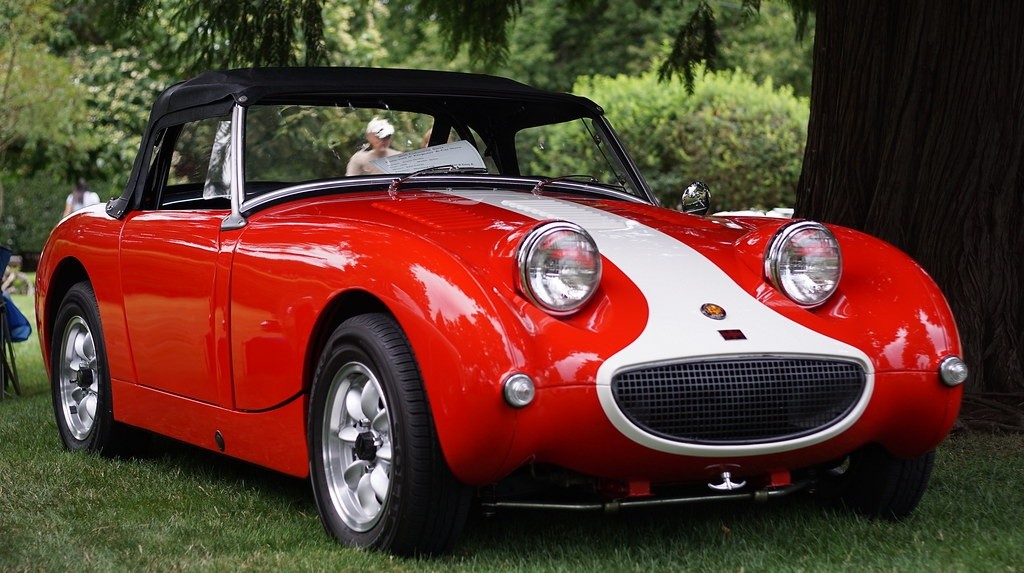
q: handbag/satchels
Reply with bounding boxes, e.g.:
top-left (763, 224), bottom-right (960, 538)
top-left (0, 292), bottom-right (32, 342)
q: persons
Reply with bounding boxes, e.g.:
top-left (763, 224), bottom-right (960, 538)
top-left (63, 177), bottom-right (100, 219)
top-left (344, 118), bottom-right (403, 176)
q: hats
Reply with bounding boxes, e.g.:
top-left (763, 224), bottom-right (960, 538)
top-left (365, 117), bottom-right (394, 139)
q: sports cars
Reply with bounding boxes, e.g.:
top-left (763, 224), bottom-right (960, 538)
top-left (32, 67), bottom-right (968, 562)
